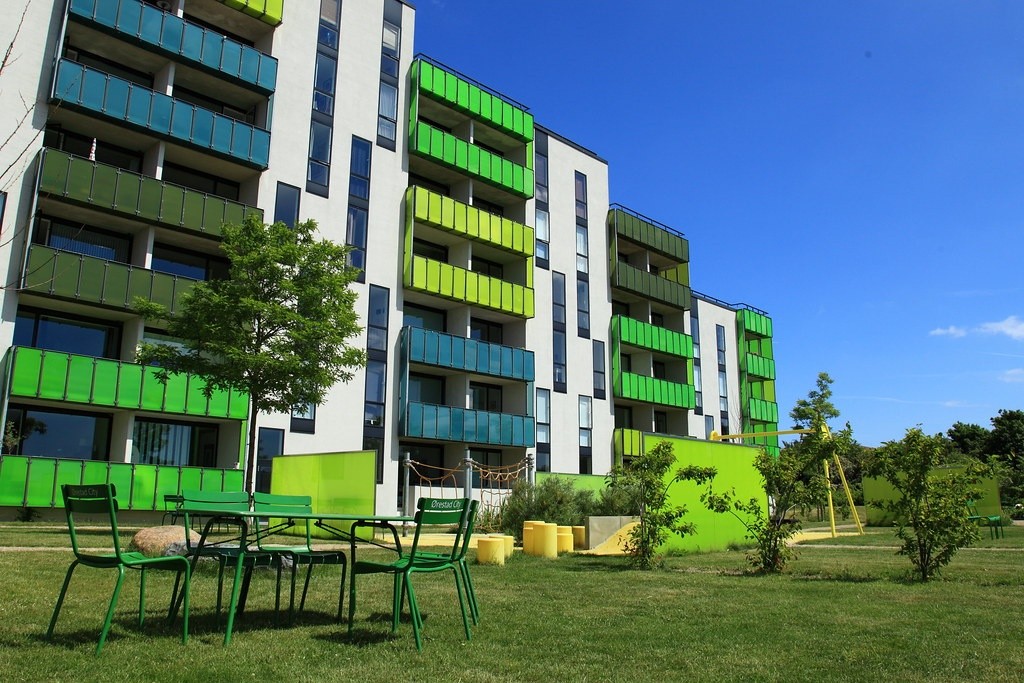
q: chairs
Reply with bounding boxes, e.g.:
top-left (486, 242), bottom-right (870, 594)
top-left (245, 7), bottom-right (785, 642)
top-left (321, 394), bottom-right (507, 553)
top-left (161, 495), bottom-right (194, 530)
top-left (347, 497), bottom-right (470, 654)
top-left (253, 492), bottom-right (346, 622)
top-left (167, 492), bottom-right (283, 625)
top-left (400, 500), bottom-right (479, 626)
top-left (46, 484), bottom-right (190, 659)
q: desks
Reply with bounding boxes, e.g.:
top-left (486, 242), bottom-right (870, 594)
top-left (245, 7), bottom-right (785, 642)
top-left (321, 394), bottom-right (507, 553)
top-left (175, 508), bottom-right (414, 647)
